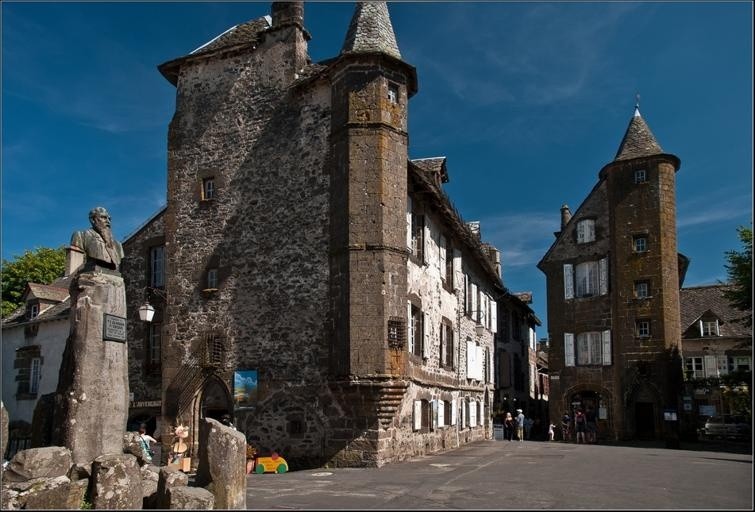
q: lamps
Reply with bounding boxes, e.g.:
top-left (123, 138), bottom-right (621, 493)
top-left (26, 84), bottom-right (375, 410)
top-left (137, 287), bottom-right (167, 323)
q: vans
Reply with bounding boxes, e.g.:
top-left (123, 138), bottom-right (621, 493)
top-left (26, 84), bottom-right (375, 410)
top-left (697, 412), bottom-right (753, 443)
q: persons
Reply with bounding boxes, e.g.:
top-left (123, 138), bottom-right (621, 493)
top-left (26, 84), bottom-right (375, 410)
top-left (560, 410), bottom-right (573, 442)
top-left (137, 428), bottom-right (157, 472)
top-left (523, 413), bottom-right (535, 441)
top-left (503, 411), bottom-right (512, 441)
top-left (547, 419), bottom-right (557, 441)
top-left (63, 204), bottom-right (129, 276)
top-left (221, 411), bottom-right (236, 428)
top-left (585, 406), bottom-right (599, 443)
top-left (514, 409), bottom-right (526, 443)
top-left (573, 408), bottom-right (588, 443)
